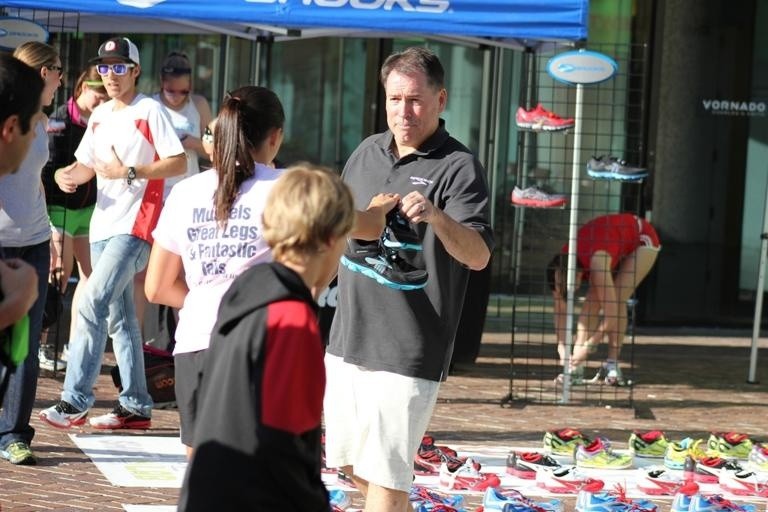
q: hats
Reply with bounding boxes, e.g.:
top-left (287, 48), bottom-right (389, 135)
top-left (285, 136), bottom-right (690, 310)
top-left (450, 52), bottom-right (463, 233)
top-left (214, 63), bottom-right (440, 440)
top-left (88, 37), bottom-right (140, 68)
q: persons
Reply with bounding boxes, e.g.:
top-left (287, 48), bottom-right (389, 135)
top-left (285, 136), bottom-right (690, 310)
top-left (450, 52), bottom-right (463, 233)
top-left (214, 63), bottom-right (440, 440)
top-left (546, 211), bottom-right (662, 384)
top-left (1, 36), bottom-right (219, 463)
top-left (176, 167), bottom-right (354, 511)
top-left (321, 47), bottom-right (495, 510)
top-left (142, 87), bottom-right (403, 460)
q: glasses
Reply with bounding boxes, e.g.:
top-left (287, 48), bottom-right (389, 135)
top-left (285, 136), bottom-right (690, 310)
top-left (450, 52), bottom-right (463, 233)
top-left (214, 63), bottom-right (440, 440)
top-left (95, 62), bottom-right (137, 77)
top-left (47, 65), bottom-right (63, 78)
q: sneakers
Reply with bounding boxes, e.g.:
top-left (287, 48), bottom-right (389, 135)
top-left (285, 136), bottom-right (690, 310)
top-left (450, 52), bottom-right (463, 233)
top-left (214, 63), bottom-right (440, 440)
top-left (586, 153), bottom-right (650, 183)
top-left (590, 482), bottom-right (657, 511)
top-left (483, 486), bottom-right (557, 512)
top-left (411, 435), bottom-right (500, 512)
top-left (544, 430), bottom-right (588, 456)
top-left (38, 401), bottom-right (88, 429)
top-left (379, 196), bottom-right (424, 252)
top-left (510, 184), bottom-right (570, 210)
top-left (338, 233), bottom-right (431, 292)
top-left (574, 437), bottom-right (633, 469)
top-left (550, 363), bottom-right (583, 388)
top-left (514, 101), bottom-right (576, 134)
top-left (89, 405), bottom-right (152, 429)
top-left (536, 466), bottom-right (605, 495)
top-left (627, 430), bottom-right (768, 509)
top-left (319, 429), bottom-right (365, 511)
top-left (0, 438), bottom-right (35, 467)
top-left (506, 450), bottom-right (562, 478)
top-left (599, 361), bottom-right (624, 386)
